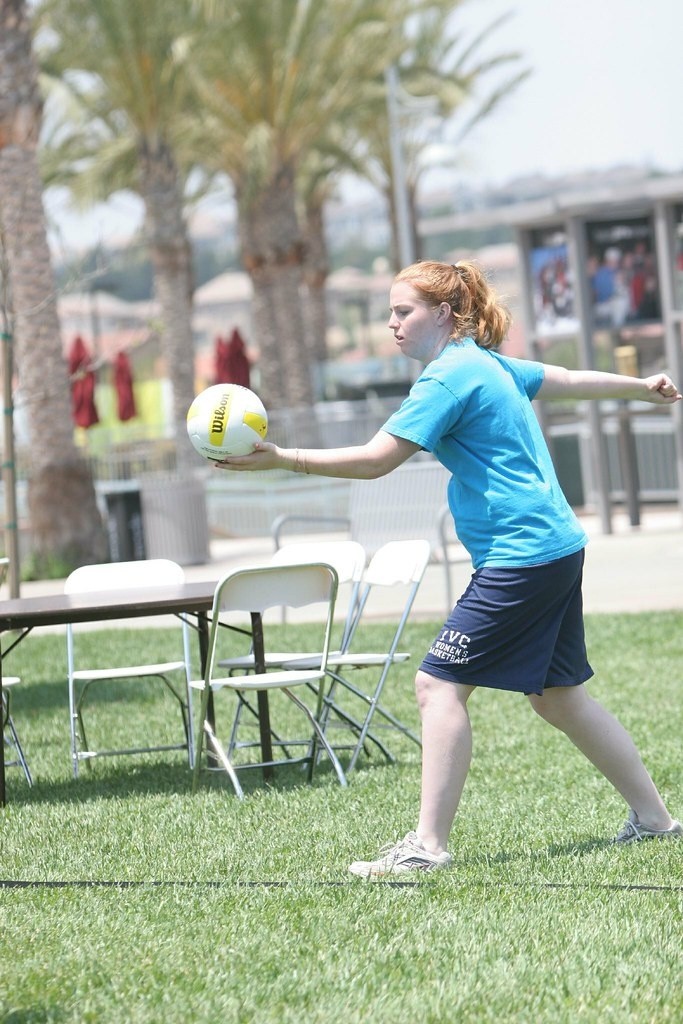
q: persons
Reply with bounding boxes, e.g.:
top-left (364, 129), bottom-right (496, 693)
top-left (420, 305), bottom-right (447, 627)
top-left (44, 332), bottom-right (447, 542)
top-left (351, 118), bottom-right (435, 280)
top-left (214, 260), bottom-right (683, 876)
top-left (586, 243), bottom-right (660, 328)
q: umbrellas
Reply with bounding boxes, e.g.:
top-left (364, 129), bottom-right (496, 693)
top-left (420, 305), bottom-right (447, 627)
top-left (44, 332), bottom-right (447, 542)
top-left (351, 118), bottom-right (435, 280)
top-left (67, 336), bottom-right (99, 447)
top-left (113, 353), bottom-right (136, 423)
top-left (214, 330), bottom-right (253, 389)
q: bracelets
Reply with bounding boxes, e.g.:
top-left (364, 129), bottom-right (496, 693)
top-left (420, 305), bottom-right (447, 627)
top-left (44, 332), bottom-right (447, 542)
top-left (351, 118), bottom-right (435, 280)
top-left (294, 447), bottom-right (310, 474)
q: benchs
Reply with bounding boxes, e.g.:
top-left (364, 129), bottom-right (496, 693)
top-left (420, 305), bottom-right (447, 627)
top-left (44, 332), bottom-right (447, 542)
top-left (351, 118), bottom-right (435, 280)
top-left (270, 461), bottom-right (458, 615)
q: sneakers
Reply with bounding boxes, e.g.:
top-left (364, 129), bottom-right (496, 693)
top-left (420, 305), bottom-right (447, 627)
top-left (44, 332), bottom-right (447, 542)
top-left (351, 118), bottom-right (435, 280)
top-left (347, 832), bottom-right (451, 877)
top-left (611, 808), bottom-right (683, 845)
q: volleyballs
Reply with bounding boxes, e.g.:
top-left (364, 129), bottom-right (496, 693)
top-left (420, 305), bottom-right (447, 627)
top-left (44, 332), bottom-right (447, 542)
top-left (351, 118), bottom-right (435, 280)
top-left (184, 383), bottom-right (271, 463)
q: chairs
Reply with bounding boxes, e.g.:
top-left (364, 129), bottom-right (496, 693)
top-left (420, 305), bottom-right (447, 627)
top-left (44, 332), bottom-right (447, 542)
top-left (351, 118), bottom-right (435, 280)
top-left (61, 538), bottom-right (431, 798)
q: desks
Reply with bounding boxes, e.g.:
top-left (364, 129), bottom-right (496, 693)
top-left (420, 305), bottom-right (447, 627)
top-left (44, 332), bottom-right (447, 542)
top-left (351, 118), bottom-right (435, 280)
top-left (0, 581), bottom-right (271, 807)
